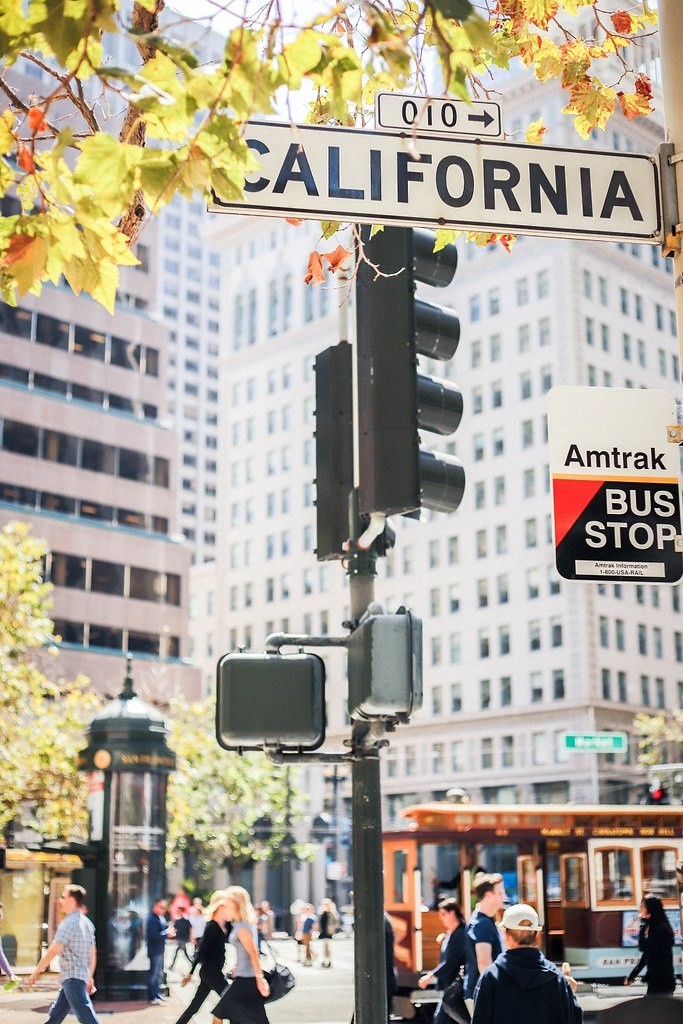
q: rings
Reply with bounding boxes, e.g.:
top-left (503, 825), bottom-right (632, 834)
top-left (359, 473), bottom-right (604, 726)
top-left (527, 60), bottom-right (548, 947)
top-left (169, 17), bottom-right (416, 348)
top-left (185, 977), bottom-right (186, 979)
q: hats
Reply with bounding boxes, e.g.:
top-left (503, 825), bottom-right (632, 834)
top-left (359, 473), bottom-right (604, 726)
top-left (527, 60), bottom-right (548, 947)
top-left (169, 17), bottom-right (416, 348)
top-left (498, 904), bottom-right (543, 931)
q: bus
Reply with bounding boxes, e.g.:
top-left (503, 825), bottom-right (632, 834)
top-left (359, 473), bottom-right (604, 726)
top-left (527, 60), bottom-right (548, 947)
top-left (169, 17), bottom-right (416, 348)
top-left (379, 807), bottom-right (683, 986)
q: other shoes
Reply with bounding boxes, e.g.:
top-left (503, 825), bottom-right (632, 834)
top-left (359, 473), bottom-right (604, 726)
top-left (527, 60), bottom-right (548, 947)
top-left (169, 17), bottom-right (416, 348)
top-left (151, 996), bottom-right (168, 1005)
top-left (321, 960), bottom-right (331, 968)
top-left (301, 959), bottom-right (312, 966)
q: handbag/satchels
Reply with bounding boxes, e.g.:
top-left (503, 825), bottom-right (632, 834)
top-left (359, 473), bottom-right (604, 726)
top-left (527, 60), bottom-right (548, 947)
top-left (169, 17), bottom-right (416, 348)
top-left (260, 963), bottom-right (296, 1004)
top-left (442, 977), bottom-right (471, 1024)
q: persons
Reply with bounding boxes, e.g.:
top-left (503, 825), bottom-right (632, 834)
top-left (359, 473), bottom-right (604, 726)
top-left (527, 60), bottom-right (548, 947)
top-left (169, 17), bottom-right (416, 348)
top-left (351, 909), bottom-right (398, 1024)
top-left (291, 898), bottom-right (354, 968)
top-left (30, 884), bottom-right (103, 1024)
top-left (417, 873), bottom-right (507, 1024)
top-left (146, 899), bottom-right (178, 1004)
top-left (471, 903), bottom-right (582, 1024)
top-left (624, 894), bottom-right (677, 996)
top-left (168, 886), bottom-right (276, 1024)
top-left (107, 909), bottom-right (144, 961)
top-left (0, 904), bottom-right (24, 983)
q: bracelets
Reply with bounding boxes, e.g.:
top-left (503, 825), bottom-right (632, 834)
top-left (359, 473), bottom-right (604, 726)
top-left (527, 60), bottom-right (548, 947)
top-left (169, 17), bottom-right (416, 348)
top-left (253, 974), bottom-right (265, 978)
top-left (426, 974), bottom-right (433, 979)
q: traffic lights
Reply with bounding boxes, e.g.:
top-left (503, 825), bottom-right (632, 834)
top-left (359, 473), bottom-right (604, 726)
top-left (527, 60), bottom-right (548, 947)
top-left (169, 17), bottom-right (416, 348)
top-left (347, 605), bottom-right (425, 722)
top-left (647, 780), bottom-right (665, 806)
top-left (355, 226), bottom-right (466, 519)
